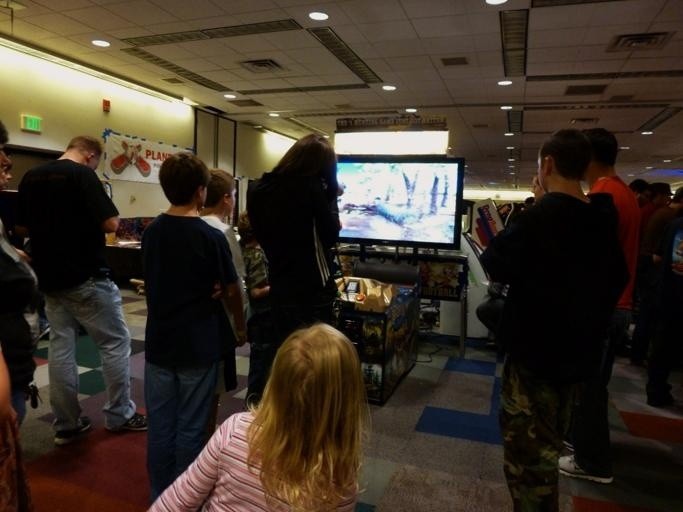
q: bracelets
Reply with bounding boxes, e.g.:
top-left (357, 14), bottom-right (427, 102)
top-left (236, 324), bottom-right (247, 338)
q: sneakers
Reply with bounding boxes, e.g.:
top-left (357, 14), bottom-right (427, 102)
top-left (646, 390), bottom-right (683, 410)
top-left (103, 412), bottom-right (147, 432)
top-left (51, 415), bottom-right (97, 446)
top-left (558, 453), bottom-right (615, 484)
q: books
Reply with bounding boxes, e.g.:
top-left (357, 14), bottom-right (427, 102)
top-left (463, 198), bottom-right (506, 283)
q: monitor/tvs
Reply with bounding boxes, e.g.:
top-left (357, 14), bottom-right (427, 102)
top-left (336, 155), bottom-right (465, 250)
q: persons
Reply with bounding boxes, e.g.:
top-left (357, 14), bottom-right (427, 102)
top-left (139, 152), bottom-right (248, 502)
top-left (197, 167), bottom-right (249, 446)
top-left (238, 133), bottom-right (343, 411)
top-left (16, 136), bottom-right (147, 446)
top-left (0, 118), bottom-right (46, 512)
top-left (234, 210), bottom-right (270, 342)
top-left (479, 128), bottom-right (683, 512)
top-left (147, 321), bottom-right (364, 510)
